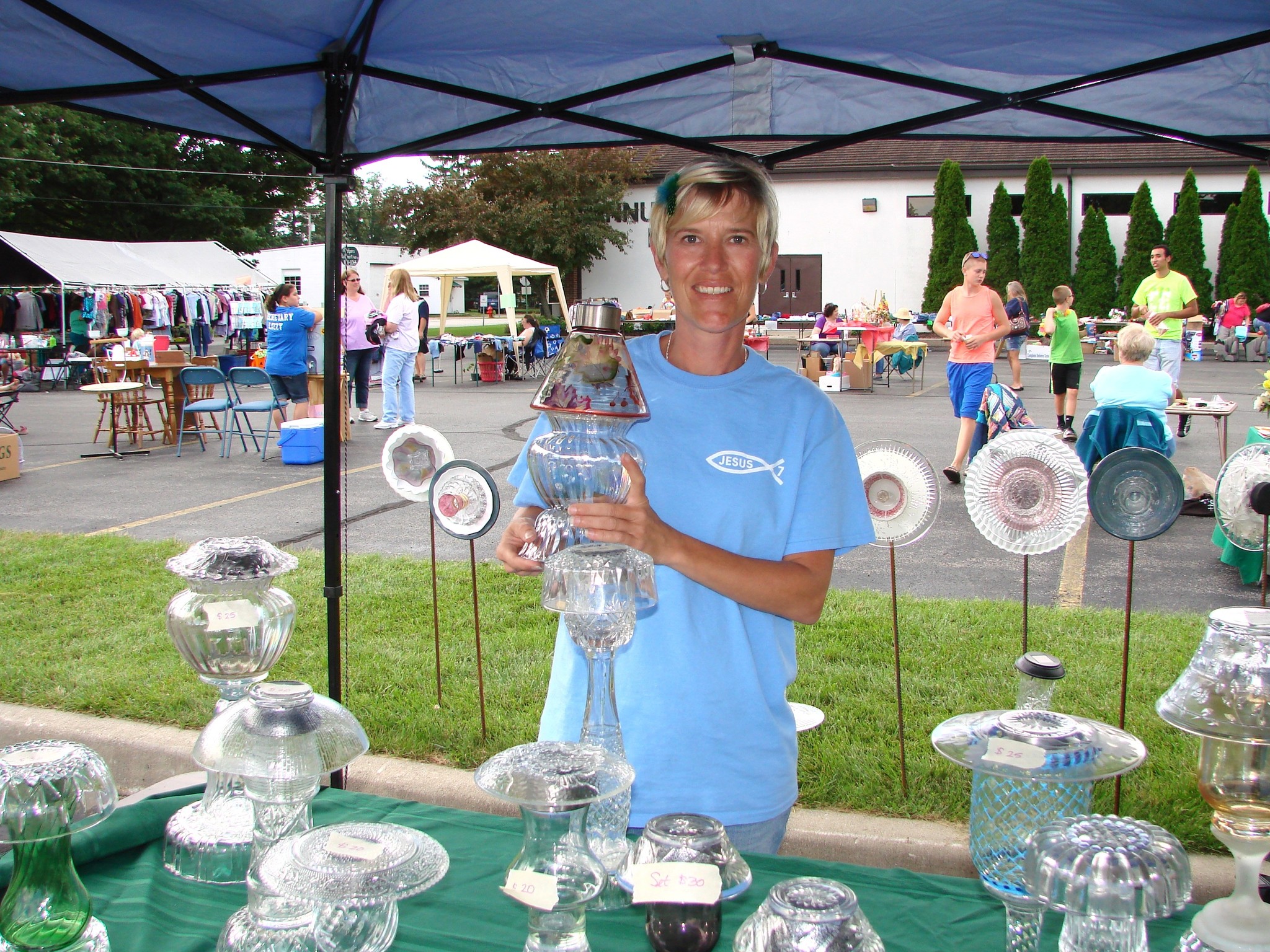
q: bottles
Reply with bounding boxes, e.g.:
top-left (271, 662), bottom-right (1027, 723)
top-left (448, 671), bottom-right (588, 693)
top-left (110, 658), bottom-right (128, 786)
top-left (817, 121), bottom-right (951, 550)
top-left (504, 301), bottom-right (719, 951)
top-left (964, 731), bottom-right (1270, 952)
top-left (165, 543), bottom-right (398, 952)
top-left (10, 336), bottom-right (16, 349)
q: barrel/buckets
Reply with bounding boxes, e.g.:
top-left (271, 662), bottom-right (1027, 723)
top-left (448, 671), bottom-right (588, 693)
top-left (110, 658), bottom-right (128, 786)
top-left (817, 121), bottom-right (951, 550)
top-left (23, 335), bottom-right (37, 348)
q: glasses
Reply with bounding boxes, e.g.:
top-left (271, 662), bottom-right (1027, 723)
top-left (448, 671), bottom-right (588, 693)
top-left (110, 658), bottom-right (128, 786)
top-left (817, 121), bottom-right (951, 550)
top-left (1065, 293), bottom-right (1075, 300)
top-left (963, 252), bottom-right (988, 267)
top-left (342, 278), bottom-right (360, 282)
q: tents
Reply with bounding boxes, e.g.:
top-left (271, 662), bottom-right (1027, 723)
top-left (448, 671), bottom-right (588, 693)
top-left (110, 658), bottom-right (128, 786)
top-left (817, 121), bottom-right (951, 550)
top-left (382, 239), bottom-right (575, 382)
top-left (1, 2), bottom-right (1266, 822)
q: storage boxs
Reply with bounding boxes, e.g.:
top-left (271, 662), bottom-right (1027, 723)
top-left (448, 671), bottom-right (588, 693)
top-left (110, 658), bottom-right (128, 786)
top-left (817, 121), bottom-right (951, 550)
top-left (622, 304), bottom-right (677, 321)
top-left (279, 416), bottom-right (329, 467)
top-left (0, 433), bottom-right (22, 484)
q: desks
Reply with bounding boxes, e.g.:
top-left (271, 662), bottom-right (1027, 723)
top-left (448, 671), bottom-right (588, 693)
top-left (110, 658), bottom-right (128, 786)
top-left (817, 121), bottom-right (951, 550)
top-left (431, 334), bottom-right (550, 386)
top-left (1025, 309), bottom-right (1267, 365)
top-left (13, 765), bottom-right (1270, 952)
top-left (1161, 399), bottom-right (1237, 465)
top-left (744, 311), bottom-right (952, 392)
top-left (302, 372), bottom-right (351, 445)
top-left (1214, 426), bottom-right (1270, 588)
top-left (620, 320), bottom-right (675, 336)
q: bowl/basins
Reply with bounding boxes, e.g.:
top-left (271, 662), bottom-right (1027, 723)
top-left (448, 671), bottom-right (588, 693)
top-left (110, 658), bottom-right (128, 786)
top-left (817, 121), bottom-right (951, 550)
top-left (1157, 606), bottom-right (1269, 748)
top-left (731, 872), bottom-right (885, 952)
top-left (614, 816), bottom-right (751, 900)
top-left (190, 682), bottom-right (367, 778)
top-left (117, 328), bottom-right (128, 337)
top-left (0, 738), bottom-right (117, 842)
top-left (88, 330), bottom-right (100, 338)
top-left (1022, 813), bottom-right (1195, 920)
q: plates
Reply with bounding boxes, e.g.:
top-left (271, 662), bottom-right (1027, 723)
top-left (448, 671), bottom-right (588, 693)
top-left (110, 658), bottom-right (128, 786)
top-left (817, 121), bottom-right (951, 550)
top-left (856, 437), bottom-right (940, 546)
top-left (1088, 446), bottom-right (1183, 539)
top-left (475, 741), bottom-right (637, 804)
top-left (963, 431), bottom-right (1089, 555)
top-left (382, 424), bottom-right (454, 503)
top-left (261, 818), bottom-right (451, 911)
top-left (426, 459), bottom-right (502, 540)
top-left (164, 535), bottom-right (298, 581)
top-left (927, 705), bottom-right (1144, 782)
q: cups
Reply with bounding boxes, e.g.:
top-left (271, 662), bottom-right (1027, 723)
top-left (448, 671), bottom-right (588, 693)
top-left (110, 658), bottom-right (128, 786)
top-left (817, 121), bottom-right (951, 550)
top-left (963, 335), bottom-right (973, 352)
top-left (1085, 322), bottom-right (1094, 338)
top-left (0, 771), bottom-right (90, 949)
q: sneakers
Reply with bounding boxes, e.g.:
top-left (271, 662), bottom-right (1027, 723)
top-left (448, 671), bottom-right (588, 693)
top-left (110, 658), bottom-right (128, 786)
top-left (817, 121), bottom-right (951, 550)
top-left (1063, 426), bottom-right (1077, 441)
top-left (1057, 423), bottom-right (1065, 431)
top-left (350, 417), bottom-right (355, 423)
top-left (373, 419), bottom-right (398, 429)
top-left (1176, 414), bottom-right (1191, 437)
top-left (397, 417), bottom-right (415, 426)
top-left (358, 409), bottom-right (378, 421)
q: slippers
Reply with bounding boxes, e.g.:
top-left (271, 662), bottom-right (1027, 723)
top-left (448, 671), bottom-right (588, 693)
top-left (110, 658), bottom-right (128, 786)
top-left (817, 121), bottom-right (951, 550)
top-left (963, 466), bottom-right (969, 476)
top-left (943, 466), bottom-right (961, 483)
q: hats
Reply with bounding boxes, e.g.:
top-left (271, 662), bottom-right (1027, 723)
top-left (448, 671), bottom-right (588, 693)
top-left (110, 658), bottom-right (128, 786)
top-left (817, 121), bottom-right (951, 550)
top-left (892, 307), bottom-right (914, 320)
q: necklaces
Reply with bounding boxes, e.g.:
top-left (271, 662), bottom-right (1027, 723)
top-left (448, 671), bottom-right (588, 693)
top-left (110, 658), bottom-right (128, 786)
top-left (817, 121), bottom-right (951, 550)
top-left (665, 330), bottom-right (748, 373)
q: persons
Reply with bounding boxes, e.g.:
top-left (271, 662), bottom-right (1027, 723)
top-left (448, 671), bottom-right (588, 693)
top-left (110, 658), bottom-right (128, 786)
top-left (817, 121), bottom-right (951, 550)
top-left (1212, 291), bottom-right (1251, 359)
top-left (340, 269), bottom-right (382, 425)
top-left (410, 287), bottom-right (431, 379)
top-left (263, 283), bottom-right (323, 434)
top-left (998, 280), bottom-right (1031, 390)
top-left (0, 336), bottom-right (11, 386)
top-left (68, 298), bottom-right (92, 384)
top-left (870, 309), bottom-right (918, 380)
top-left (504, 313), bottom-right (540, 377)
top-left (1038, 285), bottom-right (1097, 439)
top-left (379, 269), bottom-right (421, 428)
top-left (660, 292), bottom-right (674, 309)
top-left (809, 303), bottom-right (849, 361)
top-left (1089, 323), bottom-right (1179, 458)
top-left (499, 159), bottom-right (880, 854)
top-left (746, 302), bottom-right (757, 326)
top-left (1130, 245), bottom-right (1201, 437)
top-left (1253, 304), bottom-right (1270, 359)
top-left (929, 253), bottom-right (1011, 485)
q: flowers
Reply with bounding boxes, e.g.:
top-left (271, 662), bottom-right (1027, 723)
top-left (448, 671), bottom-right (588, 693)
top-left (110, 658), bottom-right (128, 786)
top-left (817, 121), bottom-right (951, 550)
top-left (1251, 368), bottom-right (1270, 420)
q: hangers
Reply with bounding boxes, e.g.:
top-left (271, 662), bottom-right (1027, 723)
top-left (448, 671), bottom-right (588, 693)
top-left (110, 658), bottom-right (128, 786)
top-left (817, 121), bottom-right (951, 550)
top-left (0, 284), bottom-right (277, 301)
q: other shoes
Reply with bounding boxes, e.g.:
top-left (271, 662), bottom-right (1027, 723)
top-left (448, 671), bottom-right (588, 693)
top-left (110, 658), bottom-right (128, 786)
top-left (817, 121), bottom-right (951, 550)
top-left (873, 374), bottom-right (882, 380)
top-left (413, 374), bottom-right (420, 377)
top-left (420, 374), bottom-right (427, 379)
top-left (1021, 387), bottom-right (1024, 390)
top-left (1008, 386), bottom-right (1021, 391)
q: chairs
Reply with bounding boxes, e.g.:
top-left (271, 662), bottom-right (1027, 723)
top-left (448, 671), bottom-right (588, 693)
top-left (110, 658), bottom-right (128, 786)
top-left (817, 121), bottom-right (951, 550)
top-left (0, 334), bottom-right (221, 459)
top-left (538, 325), bottom-right (564, 370)
top-left (1082, 405), bottom-right (1168, 470)
top-left (175, 366), bottom-right (248, 458)
top-left (223, 366), bottom-right (290, 462)
top-left (981, 384), bottom-right (1063, 440)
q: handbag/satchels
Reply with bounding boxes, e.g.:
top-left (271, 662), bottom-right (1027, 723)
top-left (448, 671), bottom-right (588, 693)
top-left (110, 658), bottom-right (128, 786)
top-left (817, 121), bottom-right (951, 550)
top-left (1009, 297), bottom-right (1028, 334)
